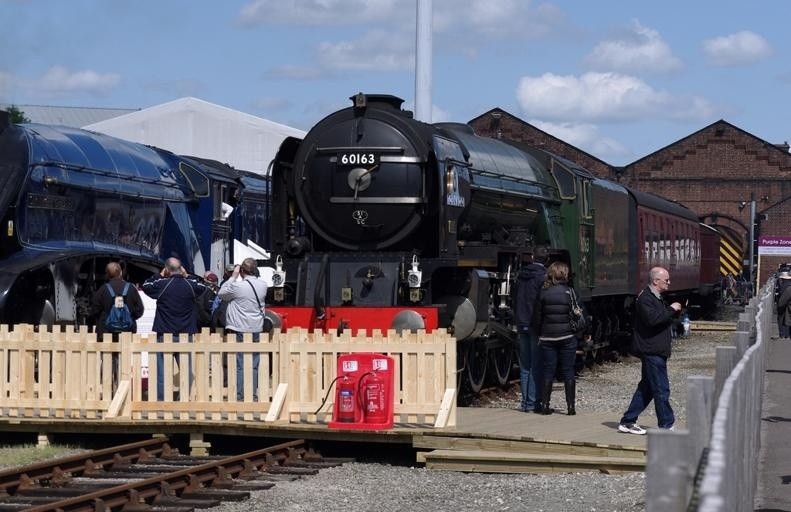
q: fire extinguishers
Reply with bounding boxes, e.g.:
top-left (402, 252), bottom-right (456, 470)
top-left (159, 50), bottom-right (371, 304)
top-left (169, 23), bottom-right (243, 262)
top-left (338, 367), bottom-right (388, 423)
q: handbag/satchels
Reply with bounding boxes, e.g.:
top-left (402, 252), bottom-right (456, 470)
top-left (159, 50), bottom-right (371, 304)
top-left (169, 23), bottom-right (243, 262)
top-left (569, 309), bottom-right (592, 333)
top-left (263, 316), bottom-right (273, 333)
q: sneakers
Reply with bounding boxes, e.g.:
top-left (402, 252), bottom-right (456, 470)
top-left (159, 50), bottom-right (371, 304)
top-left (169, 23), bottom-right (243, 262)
top-left (618, 422), bottom-right (647, 434)
top-left (522, 408), bottom-right (540, 413)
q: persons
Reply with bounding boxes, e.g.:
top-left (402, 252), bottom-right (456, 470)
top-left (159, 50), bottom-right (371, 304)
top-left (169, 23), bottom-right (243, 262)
top-left (772, 263), bottom-right (791, 340)
top-left (93, 261), bottom-right (146, 388)
top-left (718, 269), bottom-right (759, 308)
top-left (616, 266), bottom-right (684, 436)
top-left (512, 245), bottom-right (555, 411)
top-left (197, 257), bottom-right (270, 404)
top-left (530, 259), bottom-right (595, 415)
top-left (142, 256), bottom-right (212, 401)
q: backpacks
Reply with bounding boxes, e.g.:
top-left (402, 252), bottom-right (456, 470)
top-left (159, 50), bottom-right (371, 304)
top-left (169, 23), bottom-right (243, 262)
top-left (98, 282), bottom-right (133, 341)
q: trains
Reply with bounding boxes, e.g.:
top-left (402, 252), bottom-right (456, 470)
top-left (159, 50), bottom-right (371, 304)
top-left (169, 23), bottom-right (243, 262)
top-left (264, 90), bottom-right (746, 407)
top-left (2, 120), bottom-right (271, 333)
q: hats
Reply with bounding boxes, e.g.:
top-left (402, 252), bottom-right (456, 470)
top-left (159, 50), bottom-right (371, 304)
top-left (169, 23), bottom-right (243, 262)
top-left (207, 273), bottom-right (218, 282)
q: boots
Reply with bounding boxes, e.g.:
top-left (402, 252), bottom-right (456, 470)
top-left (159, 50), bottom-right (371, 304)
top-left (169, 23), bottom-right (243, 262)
top-left (541, 378), bottom-right (555, 415)
top-left (565, 379), bottom-right (576, 415)
